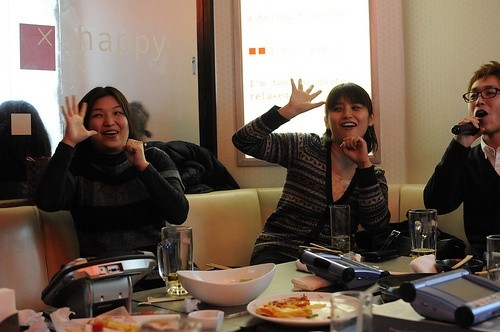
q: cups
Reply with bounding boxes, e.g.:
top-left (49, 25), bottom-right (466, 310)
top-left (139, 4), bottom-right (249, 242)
top-left (330, 291), bottom-right (373, 332)
top-left (408, 209), bottom-right (437, 262)
top-left (330, 205), bottom-right (351, 253)
top-left (486, 235), bottom-right (500, 283)
top-left (156, 226), bottom-right (194, 296)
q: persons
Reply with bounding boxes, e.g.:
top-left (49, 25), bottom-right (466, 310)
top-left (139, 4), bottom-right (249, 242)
top-left (422, 62), bottom-right (500, 265)
top-left (34, 87), bottom-right (199, 293)
top-left (232, 76), bottom-right (391, 266)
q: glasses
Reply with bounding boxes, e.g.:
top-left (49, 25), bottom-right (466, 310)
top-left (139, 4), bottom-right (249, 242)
top-left (462, 87), bottom-right (500, 104)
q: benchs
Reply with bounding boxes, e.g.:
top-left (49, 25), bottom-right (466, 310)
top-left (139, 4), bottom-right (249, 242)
top-left (0, 184), bottom-right (468, 312)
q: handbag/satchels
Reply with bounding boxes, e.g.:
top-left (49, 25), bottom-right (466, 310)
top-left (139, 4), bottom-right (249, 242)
top-left (350, 217), bottom-right (468, 263)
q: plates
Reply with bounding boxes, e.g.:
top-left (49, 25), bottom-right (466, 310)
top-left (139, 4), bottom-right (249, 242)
top-left (246, 291), bottom-right (360, 326)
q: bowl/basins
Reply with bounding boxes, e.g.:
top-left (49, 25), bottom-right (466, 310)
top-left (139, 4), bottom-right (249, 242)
top-left (434, 258), bottom-right (484, 275)
top-left (188, 309), bottom-right (224, 332)
top-left (377, 272), bottom-right (437, 304)
top-left (176, 262), bottom-right (276, 307)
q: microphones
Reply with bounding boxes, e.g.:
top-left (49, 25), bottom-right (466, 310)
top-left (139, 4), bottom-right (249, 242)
top-left (452, 122), bottom-right (480, 136)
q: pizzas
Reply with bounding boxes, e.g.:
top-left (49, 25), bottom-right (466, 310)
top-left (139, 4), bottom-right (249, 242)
top-left (256, 294), bottom-right (312, 317)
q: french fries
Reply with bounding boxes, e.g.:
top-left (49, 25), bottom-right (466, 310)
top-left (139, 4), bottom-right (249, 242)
top-left (87, 318), bottom-right (140, 332)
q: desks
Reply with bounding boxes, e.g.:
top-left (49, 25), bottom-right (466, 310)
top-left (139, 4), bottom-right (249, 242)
top-left (44, 253), bottom-right (500, 332)
